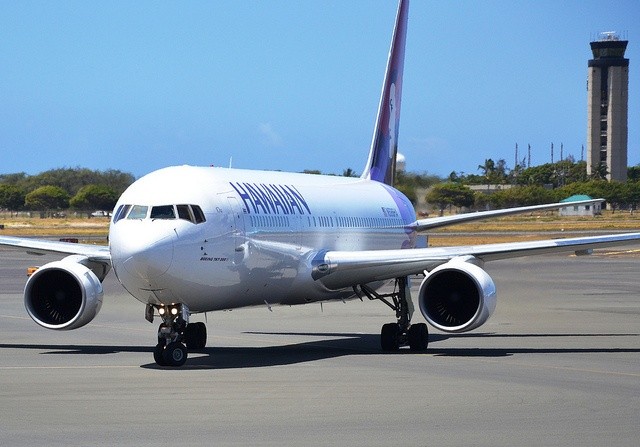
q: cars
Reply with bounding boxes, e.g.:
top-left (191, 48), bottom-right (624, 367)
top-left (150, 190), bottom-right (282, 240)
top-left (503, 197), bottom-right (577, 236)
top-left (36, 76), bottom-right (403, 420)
top-left (14, 210), bottom-right (29, 215)
top-left (90, 209), bottom-right (111, 216)
top-left (40, 210), bottom-right (65, 217)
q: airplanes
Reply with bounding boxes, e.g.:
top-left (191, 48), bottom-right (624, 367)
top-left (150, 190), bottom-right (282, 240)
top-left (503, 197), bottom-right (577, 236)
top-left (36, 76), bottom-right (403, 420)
top-left (0, 0), bottom-right (639, 365)
